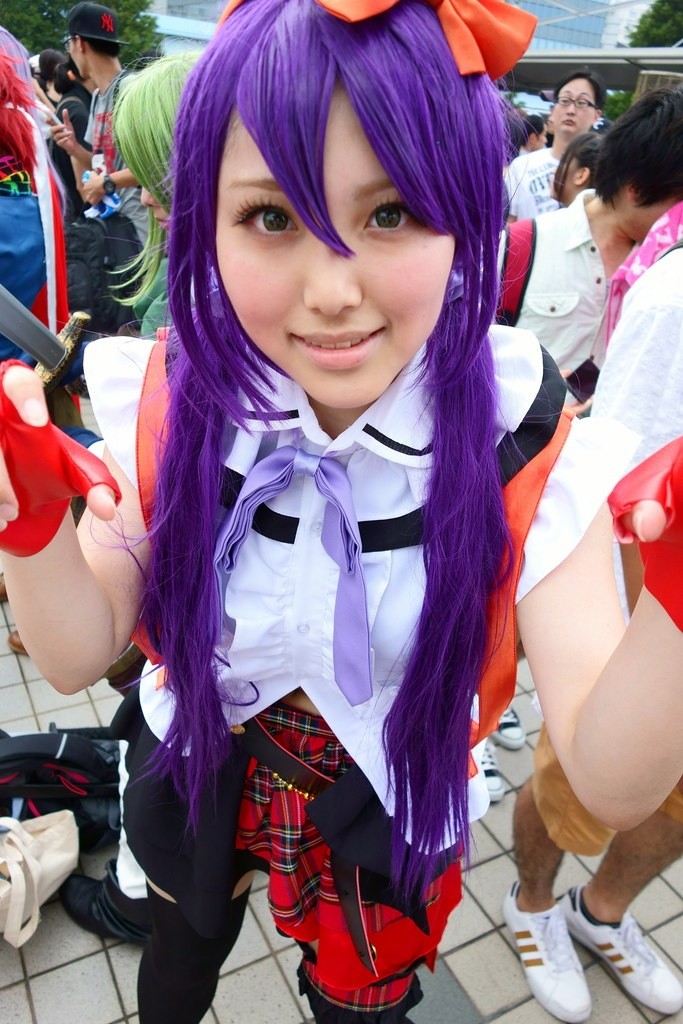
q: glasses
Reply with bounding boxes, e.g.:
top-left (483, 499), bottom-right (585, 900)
top-left (63, 35), bottom-right (84, 54)
top-left (554, 94), bottom-right (600, 111)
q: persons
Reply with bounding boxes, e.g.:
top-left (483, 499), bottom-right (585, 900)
top-left (0, 0), bottom-right (682, 1024)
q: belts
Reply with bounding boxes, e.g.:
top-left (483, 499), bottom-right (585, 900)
top-left (240, 716), bottom-right (382, 983)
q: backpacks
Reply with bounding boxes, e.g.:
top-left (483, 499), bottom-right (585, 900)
top-left (65, 211), bottom-right (144, 331)
top-left (0, 725), bottom-right (121, 854)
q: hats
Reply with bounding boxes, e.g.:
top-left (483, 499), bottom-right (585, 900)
top-left (65, 1), bottom-right (130, 45)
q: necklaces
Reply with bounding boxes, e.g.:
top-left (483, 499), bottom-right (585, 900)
top-left (91, 74), bottom-right (120, 136)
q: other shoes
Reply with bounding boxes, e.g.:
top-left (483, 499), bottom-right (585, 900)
top-left (9, 631), bottom-right (29, 653)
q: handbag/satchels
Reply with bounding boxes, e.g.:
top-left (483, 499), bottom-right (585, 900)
top-left (0, 809), bottom-right (80, 950)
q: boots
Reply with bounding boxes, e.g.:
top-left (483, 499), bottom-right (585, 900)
top-left (56, 860), bottom-right (154, 948)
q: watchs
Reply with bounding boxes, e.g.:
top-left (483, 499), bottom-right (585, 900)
top-left (103, 176), bottom-right (116, 195)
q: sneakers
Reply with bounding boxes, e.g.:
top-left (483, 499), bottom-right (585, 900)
top-left (562, 887), bottom-right (683, 1013)
top-left (502, 879), bottom-right (592, 1022)
top-left (477, 743), bottom-right (504, 800)
top-left (492, 706), bottom-right (525, 751)
